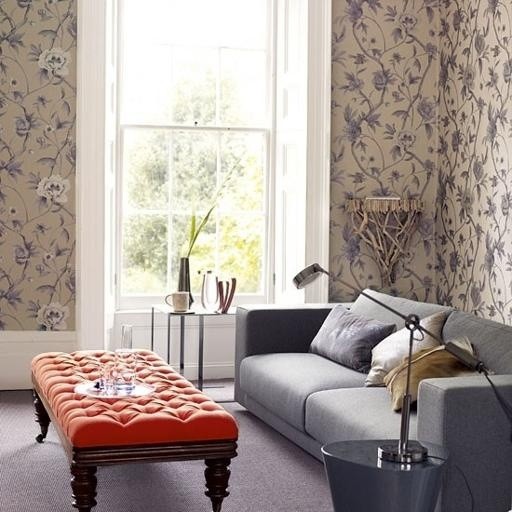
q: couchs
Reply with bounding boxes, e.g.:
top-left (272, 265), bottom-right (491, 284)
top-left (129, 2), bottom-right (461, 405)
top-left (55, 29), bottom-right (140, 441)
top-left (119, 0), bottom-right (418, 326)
top-left (234, 287), bottom-right (510, 511)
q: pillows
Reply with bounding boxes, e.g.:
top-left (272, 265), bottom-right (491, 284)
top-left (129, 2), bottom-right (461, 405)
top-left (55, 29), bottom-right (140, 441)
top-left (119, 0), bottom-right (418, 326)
top-left (307, 303), bottom-right (494, 413)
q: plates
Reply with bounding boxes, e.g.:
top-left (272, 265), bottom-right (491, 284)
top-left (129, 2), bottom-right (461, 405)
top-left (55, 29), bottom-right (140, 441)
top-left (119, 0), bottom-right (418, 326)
top-left (170, 311), bottom-right (196, 315)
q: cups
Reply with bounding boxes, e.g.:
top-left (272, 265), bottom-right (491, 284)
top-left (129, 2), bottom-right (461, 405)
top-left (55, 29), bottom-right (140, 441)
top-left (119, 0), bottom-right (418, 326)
top-left (96, 362), bottom-right (136, 393)
top-left (121, 324), bottom-right (132, 351)
top-left (165, 291), bottom-right (189, 312)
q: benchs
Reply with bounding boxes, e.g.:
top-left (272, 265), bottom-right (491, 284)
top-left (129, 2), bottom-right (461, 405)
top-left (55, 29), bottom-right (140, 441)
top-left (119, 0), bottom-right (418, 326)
top-left (31, 347), bottom-right (241, 511)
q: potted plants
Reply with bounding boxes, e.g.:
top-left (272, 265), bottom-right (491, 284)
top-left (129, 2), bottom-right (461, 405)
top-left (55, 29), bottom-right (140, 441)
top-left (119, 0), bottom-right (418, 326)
top-left (177, 203), bottom-right (217, 310)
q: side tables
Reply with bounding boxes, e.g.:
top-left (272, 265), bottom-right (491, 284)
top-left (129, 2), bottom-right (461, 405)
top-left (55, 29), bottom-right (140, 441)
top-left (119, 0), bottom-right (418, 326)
top-left (319, 439), bottom-right (450, 510)
top-left (150, 303), bottom-right (238, 404)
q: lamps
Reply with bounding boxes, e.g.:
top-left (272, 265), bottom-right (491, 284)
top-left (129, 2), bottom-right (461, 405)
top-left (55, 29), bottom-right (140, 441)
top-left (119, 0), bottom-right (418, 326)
top-left (293, 260), bottom-right (490, 465)
